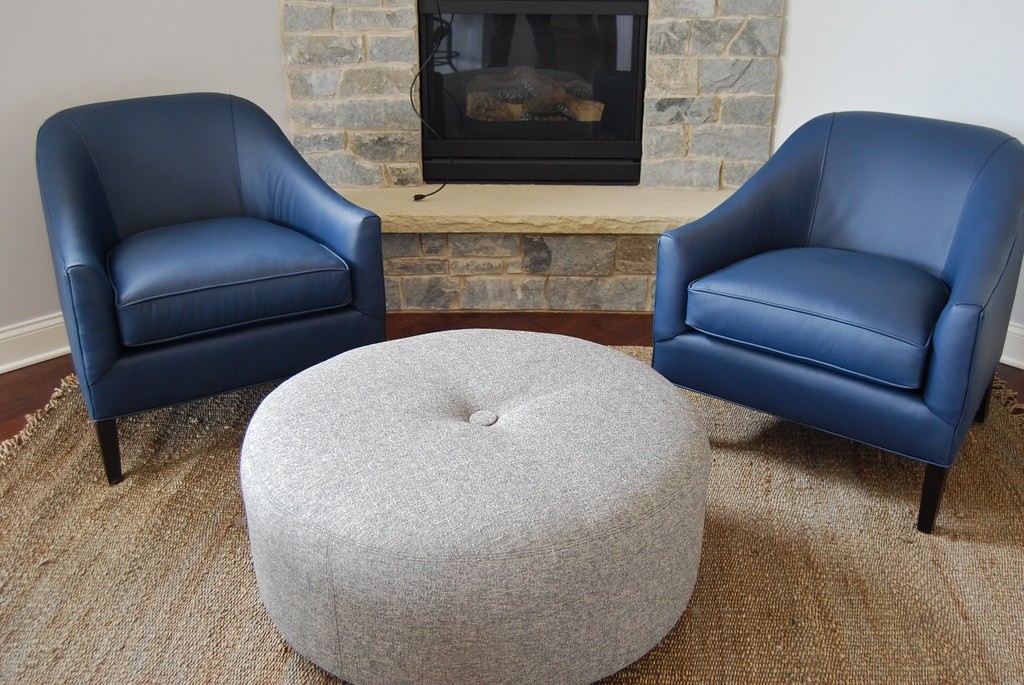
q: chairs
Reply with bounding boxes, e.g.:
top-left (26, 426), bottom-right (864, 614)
top-left (650, 112), bottom-right (1024, 534)
top-left (35, 92), bottom-right (387, 485)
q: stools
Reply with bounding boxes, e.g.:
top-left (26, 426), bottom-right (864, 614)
top-left (240, 329), bottom-right (714, 685)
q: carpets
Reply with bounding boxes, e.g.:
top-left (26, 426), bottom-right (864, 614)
top-left (0, 346), bottom-right (1024, 685)
